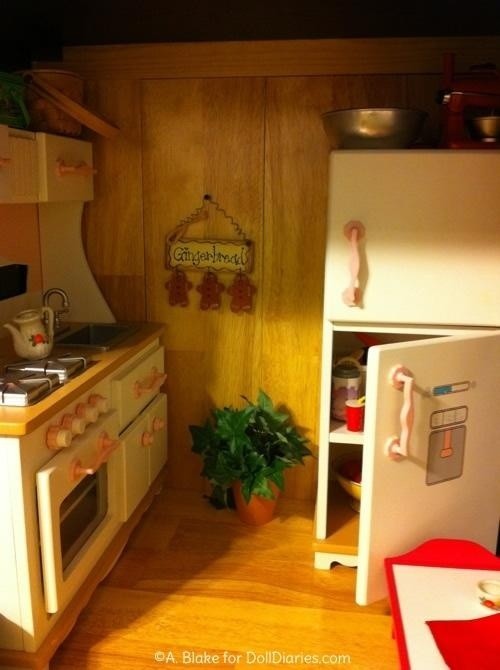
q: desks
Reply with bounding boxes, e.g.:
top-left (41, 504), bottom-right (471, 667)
top-left (384, 540), bottom-right (500, 670)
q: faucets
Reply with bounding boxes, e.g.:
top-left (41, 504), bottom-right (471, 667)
top-left (43, 288), bottom-right (69, 325)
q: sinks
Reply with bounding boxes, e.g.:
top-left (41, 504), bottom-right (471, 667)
top-left (56, 323), bottom-right (142, 351)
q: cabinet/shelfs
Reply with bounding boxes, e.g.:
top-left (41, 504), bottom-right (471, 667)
top-left (107, 336), bottom-right (168, 523)
top-left (37, 133), bottom-right (96, 202)
top-left (0, 125), bottom-right (37, 203)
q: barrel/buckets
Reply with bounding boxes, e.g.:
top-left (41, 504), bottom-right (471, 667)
top-left (330, 356), bottom-right (364, 422)
top-left (11, 57), bottom-right (87, 137)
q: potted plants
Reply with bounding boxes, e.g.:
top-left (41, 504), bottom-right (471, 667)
top-left (190, 388), bottom-right (313, 525)
